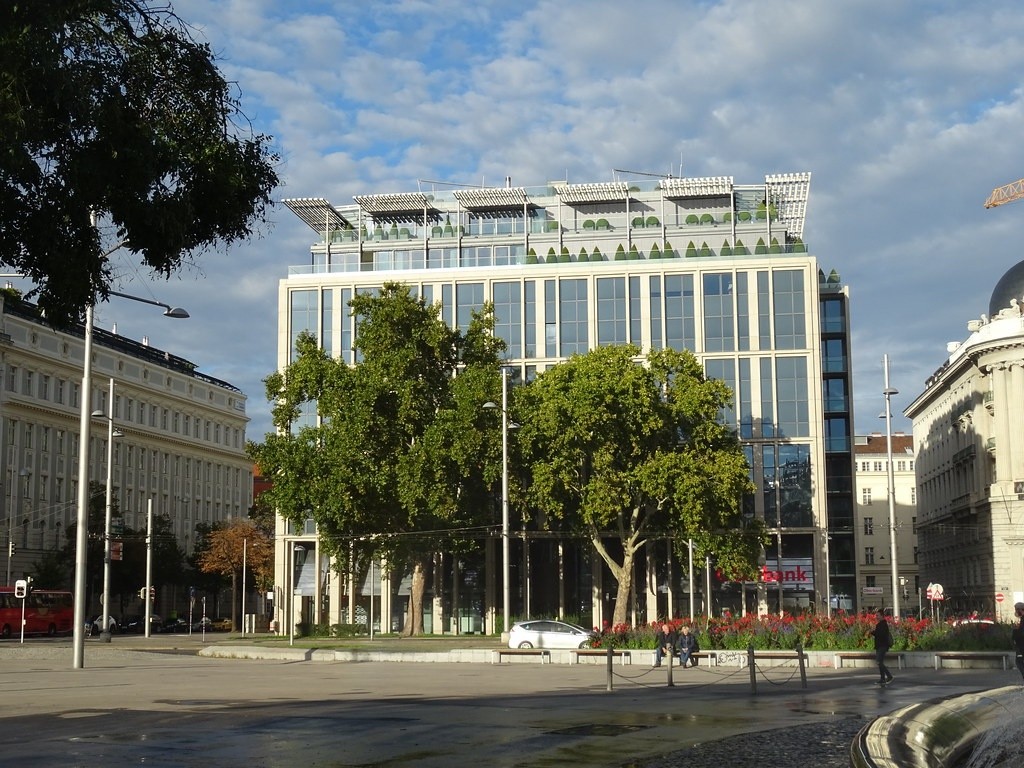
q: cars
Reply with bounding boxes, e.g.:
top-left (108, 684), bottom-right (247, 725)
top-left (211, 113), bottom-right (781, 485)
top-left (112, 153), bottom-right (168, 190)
top-left (508, 619), bottom-right (594, 651)
top-left (161, 618), bottom-right (189, 634)
top-left (91, 614), bottom-right (117, 636)
top-left (211, 617), bottom-right (232, 632)
top-left (188, 616), bottom-right (212, 632)
top-left (118, 614), bottom-right (163, 634)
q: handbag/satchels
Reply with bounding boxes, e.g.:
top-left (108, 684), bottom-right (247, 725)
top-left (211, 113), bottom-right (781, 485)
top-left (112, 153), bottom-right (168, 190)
top-left (888, 632), bottom-right (893, 647)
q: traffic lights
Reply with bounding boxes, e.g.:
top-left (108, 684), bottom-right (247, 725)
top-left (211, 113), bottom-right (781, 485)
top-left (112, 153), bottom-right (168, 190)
top-left (137, 587), bottom-right (147, 600)
top-left (26, 576), bottom-right (34, 598)
top-left (9, 542), bottom-right (15, 557)
top-left (14, 579), bottom-right (26, 598)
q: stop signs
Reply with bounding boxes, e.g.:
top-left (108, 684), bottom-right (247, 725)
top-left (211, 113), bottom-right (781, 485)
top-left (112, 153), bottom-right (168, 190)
top-left (996, 594), bottom-right (1004, 602)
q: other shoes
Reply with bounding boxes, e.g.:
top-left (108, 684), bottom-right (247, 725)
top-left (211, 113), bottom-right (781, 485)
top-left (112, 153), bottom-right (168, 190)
top-left (886, 676), bottom-right (894, 684)
top-left (653, 663), bottom-right (660, 667)
top-left (874, 680), bottom-right (887, 685)
top-left (681, 661), bottom-right (686, 667)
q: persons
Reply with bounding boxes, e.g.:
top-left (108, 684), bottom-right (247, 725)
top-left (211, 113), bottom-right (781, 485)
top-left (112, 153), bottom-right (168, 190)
top-left (679, 627), bottom-right (694, 668)
top-left (1012, 603), bottom-right (1024, 679)
top-left (654, 625), bottom-right (676, 667)
top-left (869, 608), bottom-right (894, 684)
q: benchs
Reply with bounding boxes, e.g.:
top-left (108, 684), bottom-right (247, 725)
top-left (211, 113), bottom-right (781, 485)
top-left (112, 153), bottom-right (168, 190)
top-left (569, 650), bottom-right (632, 667)
top-left (652, 652), bottom-right (718, 669)
top-left (747, 650), bottom-right (809, 668)
top-left (934, 652), bottom-right (1010, 671)
top-left (497, 649), bottom-right (552, 666)
top-left (834, 652), bottom-right (907, 670)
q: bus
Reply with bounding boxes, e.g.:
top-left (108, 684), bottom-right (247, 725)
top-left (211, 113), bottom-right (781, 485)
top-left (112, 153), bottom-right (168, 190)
top-left (0, 586), bottom-right (73, 639)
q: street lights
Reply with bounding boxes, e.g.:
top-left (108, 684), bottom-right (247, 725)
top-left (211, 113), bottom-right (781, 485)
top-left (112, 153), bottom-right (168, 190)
top-left (877, 354), bottom-right (901, 623)
top-left (241, 537), bottom-right (259, 637)
top-left (91, 378), bottom-right (125, 642)
top-left (482, 369), bottom-right (520, 644)
top-left (73, 286), bottom-right (191, 670)
